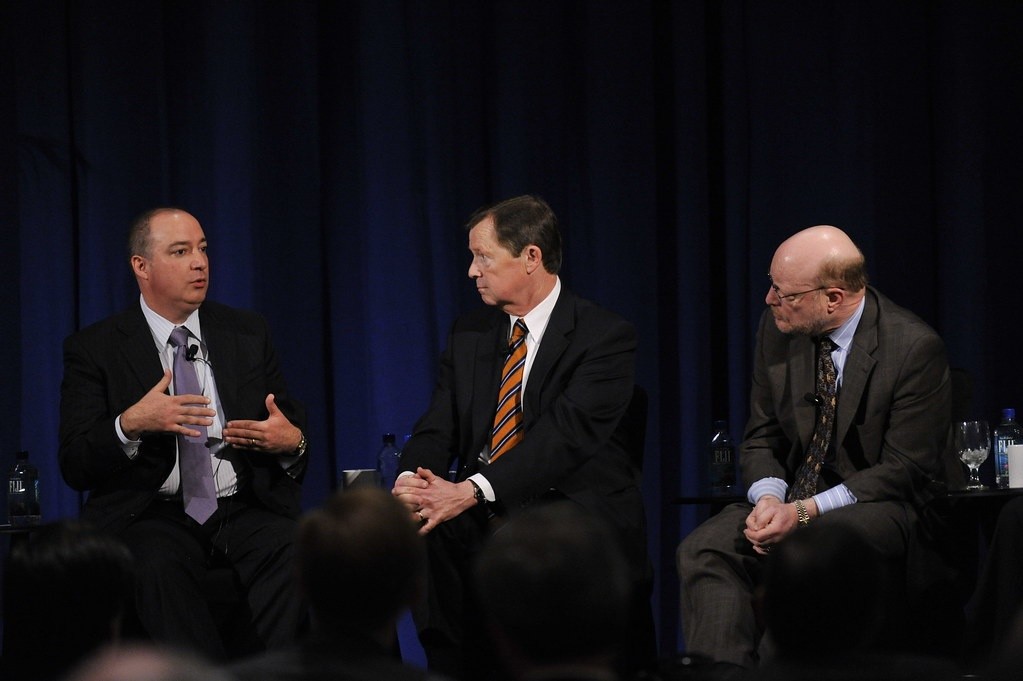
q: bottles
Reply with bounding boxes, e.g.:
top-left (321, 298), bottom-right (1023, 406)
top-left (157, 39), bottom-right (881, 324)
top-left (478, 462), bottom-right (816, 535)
top-left (7, 451), bottom-right (41, 526)
top-left (404, 434), bottom-right (411, 443)
top-left (994, 409), bottom-right (1023, 489)
top-left (378, 434), bottom-right (401, 498)
top-left (712, 421), bottom-right (737, 487)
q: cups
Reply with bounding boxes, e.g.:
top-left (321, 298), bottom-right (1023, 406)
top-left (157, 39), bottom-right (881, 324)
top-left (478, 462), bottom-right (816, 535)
top-left (343, 469), bottom-right (378, 498)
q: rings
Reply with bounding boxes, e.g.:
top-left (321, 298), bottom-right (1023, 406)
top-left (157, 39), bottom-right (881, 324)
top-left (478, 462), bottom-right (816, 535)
top-left (766, 547), bottom-right (770, 553)
top-left (419, 513), bottom-right (424, 520)
top-left (251, 439), bottom-right (255, 447)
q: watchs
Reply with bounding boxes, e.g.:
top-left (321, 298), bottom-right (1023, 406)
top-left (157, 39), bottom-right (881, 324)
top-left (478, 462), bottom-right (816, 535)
top-left (475, 487), bottom-right (484, 501)
top-left (794, 500), bottom-right (810, 529)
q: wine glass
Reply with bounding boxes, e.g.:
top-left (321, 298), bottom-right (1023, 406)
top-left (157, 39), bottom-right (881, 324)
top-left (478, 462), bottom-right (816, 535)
top-left (954, 421), bottom-right (991, 491)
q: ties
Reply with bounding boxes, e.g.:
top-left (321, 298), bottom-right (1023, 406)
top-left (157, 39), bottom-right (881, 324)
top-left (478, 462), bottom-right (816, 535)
top-left (785, 335), bottom-right (838, 504)
top-left (168, 327), bottom-right (218, 526)
top-left (491, 318), bottom-right (529, 466)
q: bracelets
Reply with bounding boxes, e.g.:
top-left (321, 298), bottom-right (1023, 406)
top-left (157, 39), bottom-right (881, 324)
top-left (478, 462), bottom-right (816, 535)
top-left (294, 435), bottom-right (307, 454)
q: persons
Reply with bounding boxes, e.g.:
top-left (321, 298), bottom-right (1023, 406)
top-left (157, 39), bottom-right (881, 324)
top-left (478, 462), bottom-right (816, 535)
top-left (390, 194), bottom-right (639, 681)
top-left (478, 500), bottom-right (619, 681)
top-left (677, 225), bottom-right (966, 681)
top-left (0, 518), bottom-right (137, 681)
top-left (58, 206), bottom-right (310, 667)
top-left (228, 486), bottom-right (432, 681)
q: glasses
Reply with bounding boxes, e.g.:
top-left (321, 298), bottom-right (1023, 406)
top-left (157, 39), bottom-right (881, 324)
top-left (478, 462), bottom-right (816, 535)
top-left (766, 271), bottom-right (847, 303)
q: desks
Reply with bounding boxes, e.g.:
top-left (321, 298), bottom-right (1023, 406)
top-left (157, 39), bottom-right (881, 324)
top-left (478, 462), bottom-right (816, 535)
top-left (0, 527), bottom-right (41, 550)
top-left (944, 487), bottom-right (1022, 565)
top-left (678, 495), bottom-right (744, 520)
